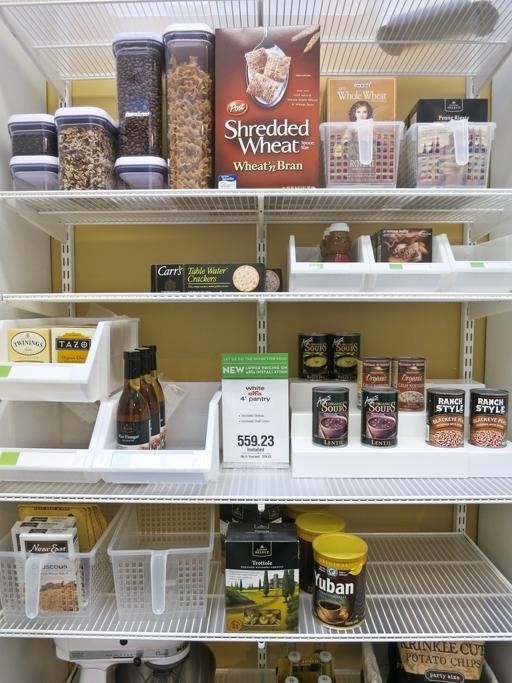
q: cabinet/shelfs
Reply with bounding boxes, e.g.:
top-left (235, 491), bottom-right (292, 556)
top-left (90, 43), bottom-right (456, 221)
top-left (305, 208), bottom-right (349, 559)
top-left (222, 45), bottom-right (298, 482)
top-left (1, 0), bottom-right (512, 683)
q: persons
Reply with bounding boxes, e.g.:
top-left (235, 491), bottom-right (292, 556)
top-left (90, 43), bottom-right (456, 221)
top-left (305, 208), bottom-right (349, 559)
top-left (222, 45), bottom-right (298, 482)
top-left (341, 102), bottom-right (380, 180)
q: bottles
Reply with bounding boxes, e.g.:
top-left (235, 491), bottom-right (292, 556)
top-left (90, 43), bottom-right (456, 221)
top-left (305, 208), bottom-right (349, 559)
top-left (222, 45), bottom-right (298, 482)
top-left (320, 222), bottom-right (352, 262)
top-left (116, 348), bottom-right (152, 450)
top-left (143, 344), bottom-right (167, 450)
top-left (135, 346), bottom-right (161, 450)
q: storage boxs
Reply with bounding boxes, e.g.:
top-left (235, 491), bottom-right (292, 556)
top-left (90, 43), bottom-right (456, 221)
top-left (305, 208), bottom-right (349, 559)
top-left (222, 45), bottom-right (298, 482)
top-left (1, 316), bottom-right (137, 404)
top-left (367, 233), bottom-right (451, 292)
top-left (1, 401), bottom-right (106, 484)
top-left (441, 234), bottom-right (512, 295)
top-left (96, 382), bottom-right (224, 480)
top-left (150, 263), bottom-right (267, 292)
top-left (288, 234), bottom-right (366, 291)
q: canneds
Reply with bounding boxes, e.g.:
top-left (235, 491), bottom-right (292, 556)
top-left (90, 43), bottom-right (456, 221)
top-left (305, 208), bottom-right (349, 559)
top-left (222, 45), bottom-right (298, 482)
top-left (312, 386), bottom-right (349, 448)
top-left (392, 356), bottom-right (427, 412)
top-left (297, 331), bottom-right (331, 381)
top-left (312, 532), bottom-right (368, 630)
top-left (470, 388), bottom-right (509, 449)
top-left (361, 385), bottom-right (397, 447)
top-left (425, 386), bottom-right (466, 448)
top-left (286, 504), bottom-right (327, 526)
top-left (355, 355), bottom-right (390, 410)
top-left (294, 514), bottom-right (348, 593)
top-left (332, 332), bottom-right (361, 381)
